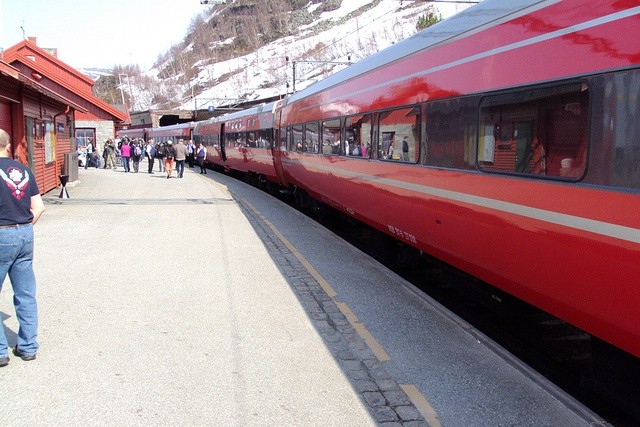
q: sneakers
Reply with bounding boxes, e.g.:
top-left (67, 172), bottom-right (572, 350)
top-left (13, 344), bottom-right (37, 360)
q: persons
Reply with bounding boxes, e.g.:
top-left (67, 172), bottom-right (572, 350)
top-left (121, 134), bottom-right (131, 140)
top-left (386, 140), bottom-right (395, 159)
top-left (187, 139), bottom-right (196, 168)
top-left (225, 133), bottom-right (386, 160)
top-left (0, 128), bottom-right (46, 366)
top-left (197, 141), bottom-right (207, 174)
top-left (131, 139), bottom-right (142, 173)
top-left (402, 135), bottom-right (410, 161)
top-left (162, 140), bottom-right (177, 179)
top-left (101, 137), bottom-right (117, 170)
top-left (174, 138), bottom-right (190, 178)
top-left (115, 135), bottom-right (121, 152)
top-left (121, 140), bottom-right (132, 173)
top-left (81, 137), bottom-right (99, 170)
top-left (137, 139), bottom-right (143, 161)
top-left (146, 139), bottom-right (156, 173)
top-left (157, 142), bottom-right (166, 172)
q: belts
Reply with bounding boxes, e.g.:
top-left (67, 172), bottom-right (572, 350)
top-left (0, 223), bottom-right (21, 229)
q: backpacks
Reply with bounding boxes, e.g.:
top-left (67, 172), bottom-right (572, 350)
top-left (165, 147), bottom-right (173, 161)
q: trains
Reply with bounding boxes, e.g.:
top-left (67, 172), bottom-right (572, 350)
top-left (119, 1), bottom-right (639, 356)
